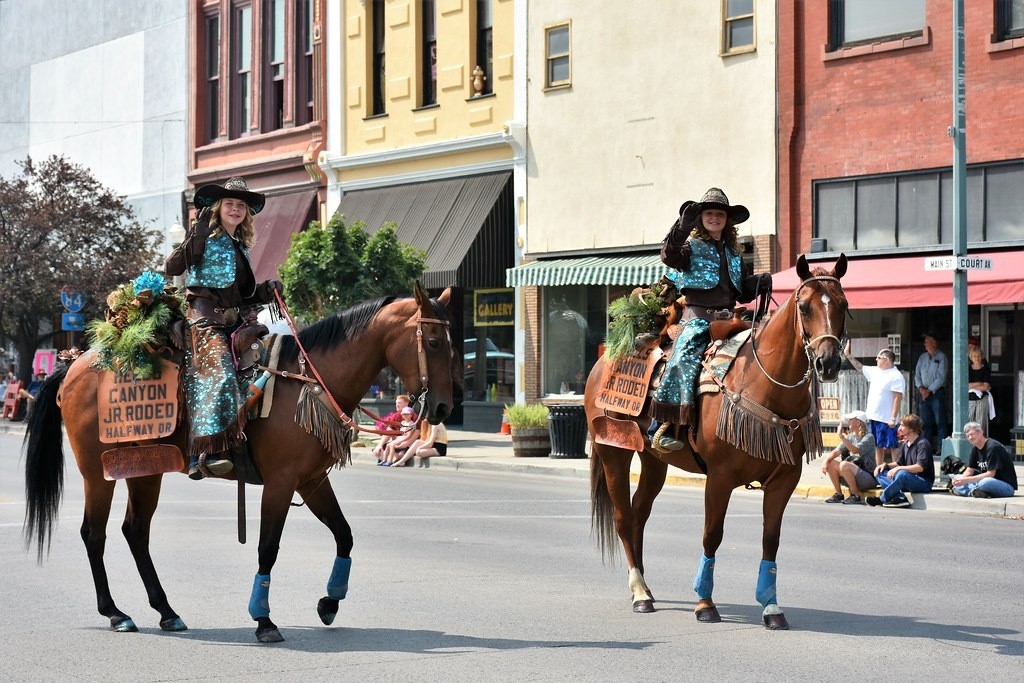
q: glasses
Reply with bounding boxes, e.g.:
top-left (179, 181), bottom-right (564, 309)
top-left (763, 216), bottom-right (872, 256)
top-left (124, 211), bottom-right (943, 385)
top-left (877, 356), bottom-right (888, 360)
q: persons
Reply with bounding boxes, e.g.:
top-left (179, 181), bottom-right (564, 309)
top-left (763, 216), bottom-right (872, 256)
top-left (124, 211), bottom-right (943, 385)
top-left (952, 423), bottom-right (1018, 498)
top-left (844, 340), bottom-right (906, 464)
top-left (374, 395), bottom-right (447, 466)
top-left (864, 414), bottom-right (935, 507)
top-left (968, 340), bottom-right (992, 427)
top-left (27, 369), bottom-right (48, 408)
top-left (648, 187), bottom-right (771, 449)
top-left (166, 177), bottom-right (283, 478)
top-left (915, 336), bottom-right (949, 454)
top-left (821, 411), bottom-right (878, 503)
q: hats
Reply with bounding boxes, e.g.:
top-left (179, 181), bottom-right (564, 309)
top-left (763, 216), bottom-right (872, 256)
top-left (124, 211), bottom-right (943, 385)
top-left (401, 407), bottom-right (413, 414)
top-left (193, 177), bottom-right (266, 216)
top-left (843, 410), bottom-right (868, 422)
top-left (679, 188), bottom-right (750, 225)
top-left (36, 369), bottom-right (47, 375)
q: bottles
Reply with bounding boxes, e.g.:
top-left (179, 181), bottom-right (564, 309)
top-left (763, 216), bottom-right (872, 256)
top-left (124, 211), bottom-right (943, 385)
top-left (491, 383), bottom-right (496, 403)
top-left (486, 384), bottom-right (491, 402)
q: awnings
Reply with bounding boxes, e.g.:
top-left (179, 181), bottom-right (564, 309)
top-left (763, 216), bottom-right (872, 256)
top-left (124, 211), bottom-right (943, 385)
top-left (249, 190), bottom-right (316, 283)
top-left (506, 255), bottom-right (676, 287)
top-left (333, 171), bottom-right (514, 289)
top-left (735, 253), bottom-right (1024, 309)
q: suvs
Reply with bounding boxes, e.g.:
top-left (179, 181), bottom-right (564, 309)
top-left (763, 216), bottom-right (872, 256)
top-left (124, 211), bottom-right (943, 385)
top-left (464, 338), bottom-right (514, 387)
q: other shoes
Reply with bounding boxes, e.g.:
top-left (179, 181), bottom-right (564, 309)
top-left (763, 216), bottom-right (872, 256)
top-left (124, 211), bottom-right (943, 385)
top-left (648, 435), bottom-right (684, 452)
top-left (377, 460), bottom-right (385, 465)
top-left (383, 462), bottom-right (393, 466)
top-left (189, 455), bottom-right (233, 480)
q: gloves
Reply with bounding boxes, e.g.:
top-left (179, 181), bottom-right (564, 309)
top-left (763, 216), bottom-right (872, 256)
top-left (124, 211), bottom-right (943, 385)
top-left (267, 280), bottom-right (284, 298)
top-left (760, 272), bottom-right (773, 291)
top-left (680, 204), bottom-right (703, 229)
top-left (192, 208), bottom-right (221, 255)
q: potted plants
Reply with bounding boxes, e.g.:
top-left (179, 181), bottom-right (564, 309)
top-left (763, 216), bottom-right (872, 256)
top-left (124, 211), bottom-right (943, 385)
top-left (504, 402), bottom-right (551, 458)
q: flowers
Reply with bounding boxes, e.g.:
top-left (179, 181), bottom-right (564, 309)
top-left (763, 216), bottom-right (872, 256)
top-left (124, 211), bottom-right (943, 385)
top-left (133, 271), bottom-right (167, 297)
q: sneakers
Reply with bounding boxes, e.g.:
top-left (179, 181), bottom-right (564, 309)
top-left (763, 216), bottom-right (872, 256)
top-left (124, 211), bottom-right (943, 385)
top-left (866, 497), bottom-right (882, 507)
top-left (883, 496), bottom-right (910, 507)
top-left (826, 493), bottom-right (845, 503)
top-left (952, 487), bottom-right (959, 496)
top-left (843, 494), bottom-right (862, 504)
top-left (972, 490), bottom-right (988, 499)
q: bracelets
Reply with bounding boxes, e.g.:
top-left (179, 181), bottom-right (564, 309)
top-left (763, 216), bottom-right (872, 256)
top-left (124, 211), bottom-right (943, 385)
top-left (891, 417), bottom-right (896, 420)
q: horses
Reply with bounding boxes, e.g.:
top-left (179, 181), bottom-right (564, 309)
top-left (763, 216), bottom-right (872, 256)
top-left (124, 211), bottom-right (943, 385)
top-left (584, 253), bottom-right (848, 630)
top-left (22, 280), bottom-right (453, 644)
